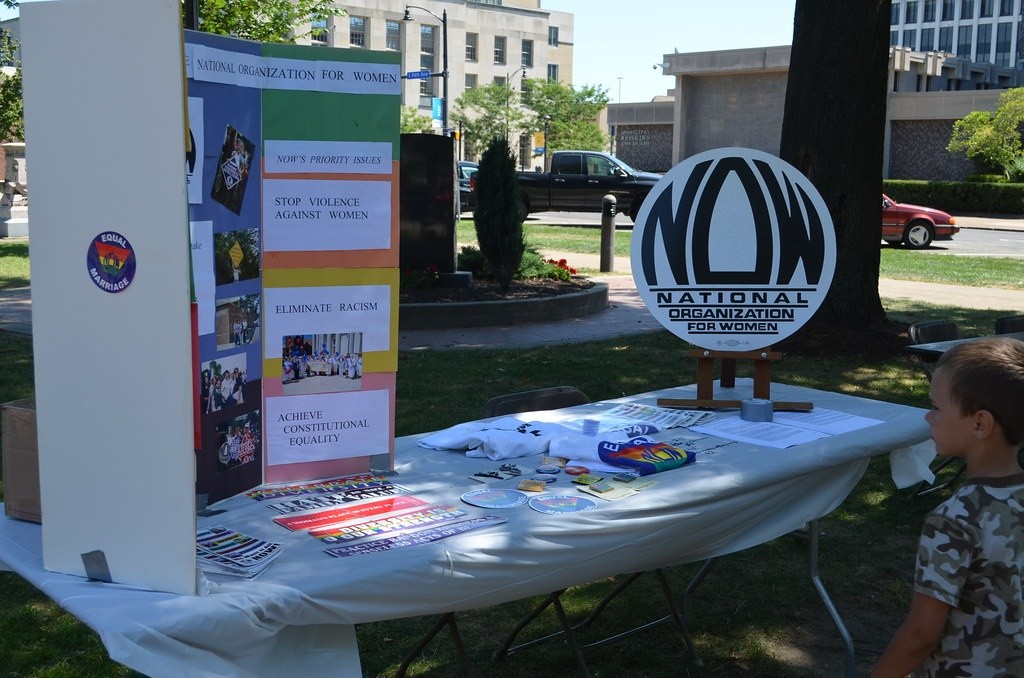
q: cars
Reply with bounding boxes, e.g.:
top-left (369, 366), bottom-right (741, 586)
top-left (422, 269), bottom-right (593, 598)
top-left (882, 194), bottom-right (961, 248)
top-left (456, 161), bottom-right (482, 211)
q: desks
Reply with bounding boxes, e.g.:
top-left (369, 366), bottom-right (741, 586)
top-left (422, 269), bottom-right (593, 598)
top-left (905, 331), bottom-right (1024, 366)
top-left (0, 378), bottom-right (937, 678)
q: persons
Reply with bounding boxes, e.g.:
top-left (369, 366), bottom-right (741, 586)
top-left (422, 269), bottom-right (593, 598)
top-left (282, 337), bottom-right (362, 383)
top-left (867, 337), bottom-right (1024, 678)
top-left (202, 368), bottom-right (244, 414)
top-left (219, 137), bottom-right (249, 200)
top-left (233, 318), bottom-right (248, 346)
top-left (216, 422), bottom-right (256, 470)
top-left (232, 263), bottom-right (241, 283)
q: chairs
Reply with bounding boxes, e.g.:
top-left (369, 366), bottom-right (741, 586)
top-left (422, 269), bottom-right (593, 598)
top-left (907, 318), bottom-right (966, 501)
top-left (393, 386), bottom-right (705, 678)
top-left (994, 315), bottom-right (1024, 335)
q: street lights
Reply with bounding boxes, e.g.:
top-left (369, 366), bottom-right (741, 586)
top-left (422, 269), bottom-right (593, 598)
top-left (506, 67), bottom-right (527, 150)
top-left (544, 114), bottom-right (551, 173)
top-left (617, 77), bottom-right (620, 104)
top-left (402, 4), bottom-right (447, 136)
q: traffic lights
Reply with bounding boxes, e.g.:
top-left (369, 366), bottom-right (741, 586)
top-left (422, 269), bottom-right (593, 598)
top-left (451, 131), bottom-right (460, 140)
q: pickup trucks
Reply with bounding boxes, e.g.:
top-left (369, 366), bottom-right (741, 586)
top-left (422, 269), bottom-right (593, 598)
top-left (466, 150), bottom-right (669, 231)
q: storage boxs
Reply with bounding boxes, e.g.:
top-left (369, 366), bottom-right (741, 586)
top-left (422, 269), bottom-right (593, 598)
top-left (0, 396), bottom-right (42, 524)
